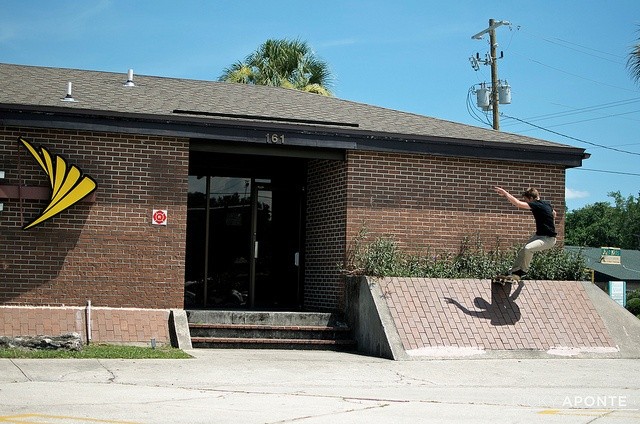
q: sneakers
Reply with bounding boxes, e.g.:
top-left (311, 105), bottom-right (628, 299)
top-left (511, 269), bottom-right (528, 277)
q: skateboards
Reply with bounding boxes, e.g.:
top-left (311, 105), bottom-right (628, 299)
top-left (490, 274), bottom-right (521, 286)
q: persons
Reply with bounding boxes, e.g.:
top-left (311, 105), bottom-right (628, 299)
top-left (494, 187), bottom-right (557, 275)
